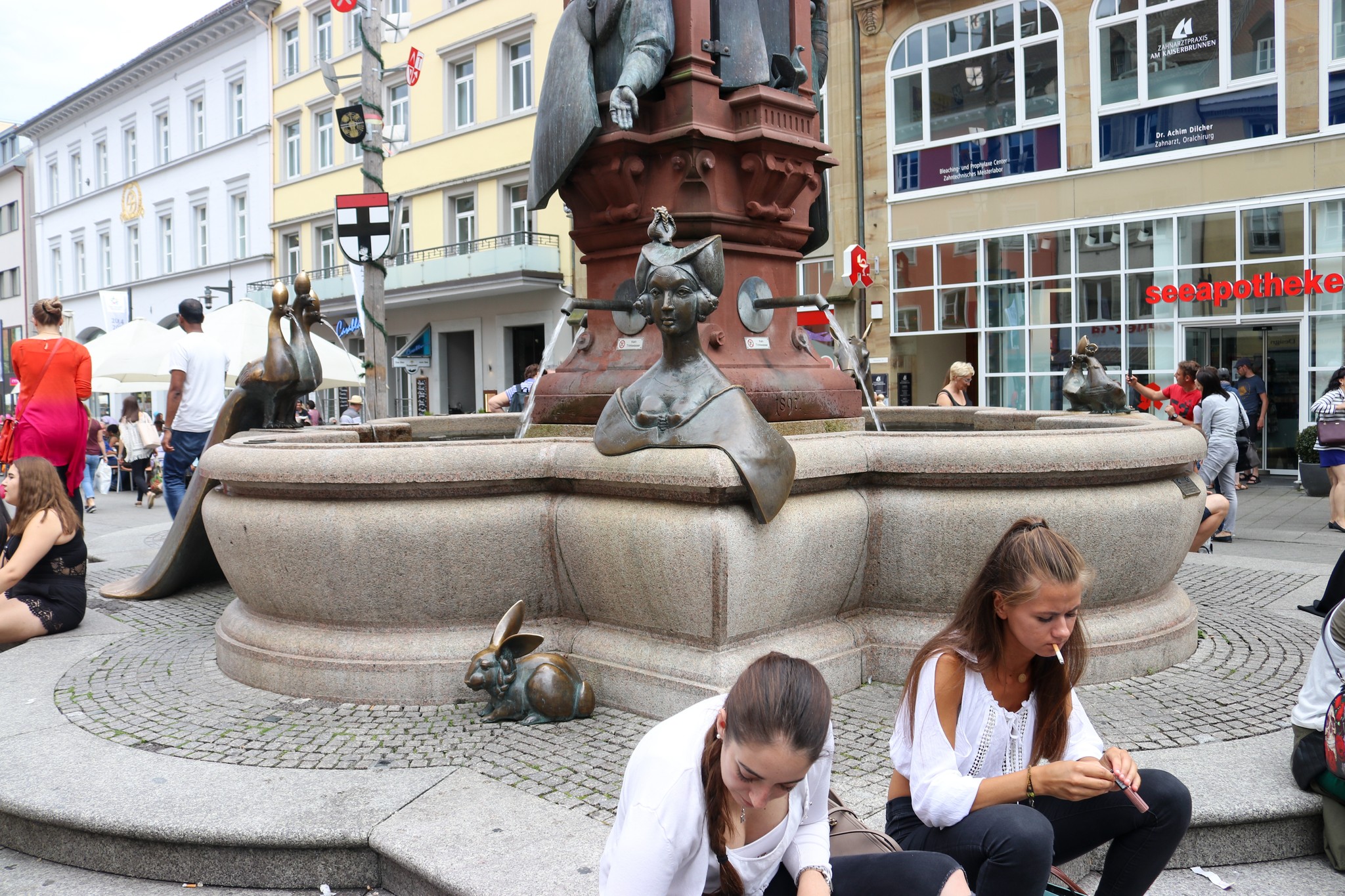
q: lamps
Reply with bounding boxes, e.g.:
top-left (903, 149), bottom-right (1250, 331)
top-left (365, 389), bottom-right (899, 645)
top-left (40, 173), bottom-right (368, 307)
top-left (1111, 231), bottom-right (1121, 244)
top-left (1040, 238), bottom-right (1051, 250)
top-left (1084, 234), bottom-right (1097, 247)
top-left (1137, 228), bottom-right (1149, 241)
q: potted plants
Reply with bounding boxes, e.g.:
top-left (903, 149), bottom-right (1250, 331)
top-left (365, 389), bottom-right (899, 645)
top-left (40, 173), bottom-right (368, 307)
top-left (1294, 425), bottom-right (1332, 498)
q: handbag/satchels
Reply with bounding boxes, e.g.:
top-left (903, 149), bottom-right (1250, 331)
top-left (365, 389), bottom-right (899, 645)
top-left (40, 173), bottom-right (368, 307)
top-left (1324, 685), bottom-right (1344, 780)
top-left (0, 418), bottom-right (14, 464)
top-left (138, 423), bottom-right (161, 449)
top-left (1317, 419), bottom-right (1345, 445)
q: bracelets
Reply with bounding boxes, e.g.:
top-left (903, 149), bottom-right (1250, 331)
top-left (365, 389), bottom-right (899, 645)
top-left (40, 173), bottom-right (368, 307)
top-left (162, 423), bottom-right (172, 432)
top-left (1026, 765), bottom-right (1035, 809)
top-left (795, 865), bottom-right (834, 896)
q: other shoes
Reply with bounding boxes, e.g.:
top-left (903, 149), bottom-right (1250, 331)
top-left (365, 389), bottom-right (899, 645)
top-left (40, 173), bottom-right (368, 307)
top-left (1328, 521), bottom-right (1345, 533)
top-left (85, 504), bottom-right (96, 513)
top-left (147, 492), bottom-right (156, 510)
top-left (135, 501), bottom-right (141, 506)
top-left (1213, 535), bottom-right (1233, 542)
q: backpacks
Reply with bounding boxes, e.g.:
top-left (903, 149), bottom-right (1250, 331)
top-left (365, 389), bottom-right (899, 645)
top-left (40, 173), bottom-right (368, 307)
top-left (509, 384), bottom-right (529, 412)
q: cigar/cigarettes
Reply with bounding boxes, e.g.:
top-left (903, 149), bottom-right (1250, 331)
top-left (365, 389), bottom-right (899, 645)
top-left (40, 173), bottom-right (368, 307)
top-left (1052, 644), bottom-right (1065, 665)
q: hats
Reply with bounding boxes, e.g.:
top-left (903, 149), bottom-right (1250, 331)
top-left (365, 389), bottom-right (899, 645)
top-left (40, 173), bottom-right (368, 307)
top-left (1217, 368), bottom-right (1230, 379)
top-left (1233, 358), bottom-right (1252, 367)
top-left (348, 395), bottom-right (364, 404)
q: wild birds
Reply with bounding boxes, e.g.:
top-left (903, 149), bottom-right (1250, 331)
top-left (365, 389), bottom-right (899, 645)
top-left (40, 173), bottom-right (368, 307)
top-left (101, 270), bottom-right (328, 601)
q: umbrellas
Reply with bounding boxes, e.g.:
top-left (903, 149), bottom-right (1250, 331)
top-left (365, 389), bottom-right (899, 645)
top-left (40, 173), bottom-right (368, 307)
top-left (92, 297), bottom-right (391, 393)
top-left (82, 316), bottom-right (187, 383)
top-left (90, 377), bottom-right (173, 393)
top-left (10, 382), bottom-right (20, 394)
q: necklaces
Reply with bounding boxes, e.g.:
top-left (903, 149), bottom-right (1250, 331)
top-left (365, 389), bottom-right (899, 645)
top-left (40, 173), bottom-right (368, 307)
top-left (39, 332), bottom-right (61, 336)
top-left (997, 658), bottom-right (1032, 684)
top-left (740, 806), bottom-right (747, 824)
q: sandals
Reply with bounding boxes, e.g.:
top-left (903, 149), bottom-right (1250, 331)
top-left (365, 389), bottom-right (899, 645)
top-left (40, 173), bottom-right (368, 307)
top-left (1235, 483), bottom-right (1248, 491)
top-left (1240, 473), bottom-right (1250, 481)
top-left (1246, 475), bottom-right (1261, 484)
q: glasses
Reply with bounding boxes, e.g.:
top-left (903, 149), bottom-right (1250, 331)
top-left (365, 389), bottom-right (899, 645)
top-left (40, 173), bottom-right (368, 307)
top-left (963, 378), bottom-right (972, 382)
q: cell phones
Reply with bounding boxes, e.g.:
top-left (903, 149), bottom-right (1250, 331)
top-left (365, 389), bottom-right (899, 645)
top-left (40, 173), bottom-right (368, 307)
top-left (1128, 367), bottom-right (1132, 382)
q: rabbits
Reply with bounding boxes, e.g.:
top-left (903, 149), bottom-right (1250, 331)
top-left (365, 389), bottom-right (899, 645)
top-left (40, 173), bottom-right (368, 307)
top-left (463, 599), bottom-right (597, 724)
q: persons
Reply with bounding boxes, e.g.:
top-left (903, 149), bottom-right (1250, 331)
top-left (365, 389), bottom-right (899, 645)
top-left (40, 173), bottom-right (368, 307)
top-left (488, 363), bottom-right (549, 413)
top-left (9, 298), bottom-right (92, 526)
top-left (1290, 599), bottom-right (1345, 872)
top-left (0, 454), bottom-right (89, 652)
top-left (935, 361), bottom-right (976, 407)
top-left (592, 206), bottom-right (796, 524)
top-left (0, 394), bottom-right (364, 553)
top-left (1125, 357), bottom-right (1277, 552)
top-left (524, 0), bottom-right (675, 212)
top-left (598, 648), bottom-right (977, 896)
top-left (160, 297), bottom-right (230, 523)
top-left (1309, 366), bottom-right (1345, 534)
top-left (884, 513), bottom-right (1194, 896)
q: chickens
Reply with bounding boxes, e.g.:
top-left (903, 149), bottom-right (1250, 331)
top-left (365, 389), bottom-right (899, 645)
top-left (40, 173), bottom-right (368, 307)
top-left (1062, 335), bottom-right (1091, 411)
top-left (1078, 343), bottom-right (1135, 415)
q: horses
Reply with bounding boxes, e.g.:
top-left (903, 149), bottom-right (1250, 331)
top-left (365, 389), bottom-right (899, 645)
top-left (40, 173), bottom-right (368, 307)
top-left (829, 321), bottom-right (876, 407)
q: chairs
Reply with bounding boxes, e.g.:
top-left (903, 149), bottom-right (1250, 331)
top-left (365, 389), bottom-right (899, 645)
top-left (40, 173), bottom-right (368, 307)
top-left (103, 454), bottom-right (134, 493)
